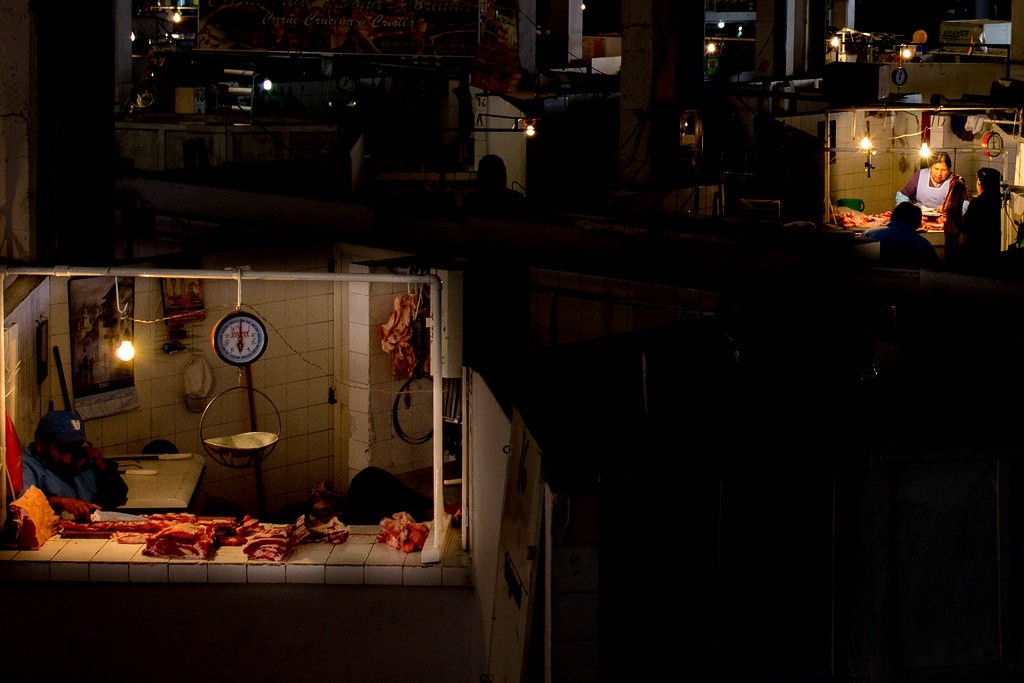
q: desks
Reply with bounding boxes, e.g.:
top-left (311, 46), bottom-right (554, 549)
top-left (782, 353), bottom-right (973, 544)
top-left (110, 453), bottom-right (208, 512)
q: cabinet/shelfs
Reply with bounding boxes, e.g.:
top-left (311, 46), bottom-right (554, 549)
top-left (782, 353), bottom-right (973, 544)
top-left (113, 116), bottom-right (338, 171)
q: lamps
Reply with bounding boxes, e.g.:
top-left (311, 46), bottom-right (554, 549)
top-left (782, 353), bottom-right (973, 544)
top-left (707, 40), bottom-right (714, 52)
top-left (262, 75), bottom-right (273, 91)
top-left (920, 131), bottom-right (931, 157)
top-left (718, 19), bottom-right (724, 29)
top-left (526, 125), bottom-right (535, 136)
top-left (902, 45), bottom-right (911, 58)
top-left (861, 137), bottom-right (872, 150)
top-left (118, 319), bottom-right (135, 361)
top-left (831, 34), bottom-right (839, 46)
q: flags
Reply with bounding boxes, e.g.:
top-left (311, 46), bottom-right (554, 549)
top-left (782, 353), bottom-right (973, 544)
top-left (5, 410), bottom-right (23, 496)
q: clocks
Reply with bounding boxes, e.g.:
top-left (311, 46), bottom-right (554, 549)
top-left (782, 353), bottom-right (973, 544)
top-left (892, 67), bottom-right (908, 86)
top-left (211, 310), bottom-right (268, 368)
top-left (338, 73), bottom-right (355, 91)
top-left (982, 131), bottom-right (1004, 157)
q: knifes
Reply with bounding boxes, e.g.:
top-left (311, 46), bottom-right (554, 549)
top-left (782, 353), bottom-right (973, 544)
top-left (106, 453), bottom-right (192, 462)
top-left (119, 468), bottom-right (158, 475)
top-left (61, 510), bottom-right (149, 523)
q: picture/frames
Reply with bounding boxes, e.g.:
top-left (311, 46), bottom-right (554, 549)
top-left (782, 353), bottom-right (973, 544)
top-left (159, 278), bottom-right (207, 328)
top-left (67, 276), bottom-right (141, 422)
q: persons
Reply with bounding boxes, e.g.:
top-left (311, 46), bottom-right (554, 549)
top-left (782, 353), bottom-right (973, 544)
top-left (946, 168), bottom-right (1004, 279)
top-left (16, 410), bottom-right (129, 524)
top-left (860, 202), bottom-right (943, 273)
top-left (899, 152), bottom-right (967, 223)
top-left (462, 155), bottom-right (535, 223)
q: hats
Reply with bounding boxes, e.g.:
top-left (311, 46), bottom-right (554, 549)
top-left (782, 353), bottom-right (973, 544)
top-left (36, 410), bottom-right (86, 443)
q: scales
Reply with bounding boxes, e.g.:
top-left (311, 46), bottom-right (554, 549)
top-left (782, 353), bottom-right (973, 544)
top-left (198, 310), bottom-right (281, 468)
